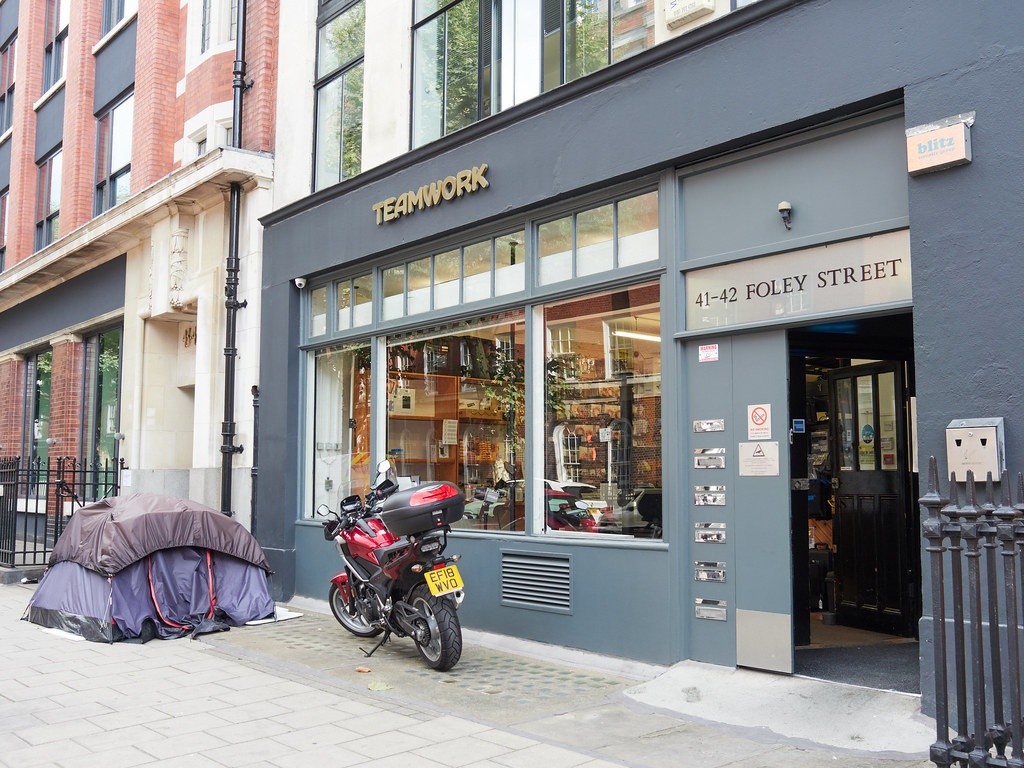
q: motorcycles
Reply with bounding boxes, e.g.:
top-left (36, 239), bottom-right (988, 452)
top-left (315, 461), bottom-right (465, 673)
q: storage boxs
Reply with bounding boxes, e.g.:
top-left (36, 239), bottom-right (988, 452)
top-left (382, 482), bottom-right (467, 535)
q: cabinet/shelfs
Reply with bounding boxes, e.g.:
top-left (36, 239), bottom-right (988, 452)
top-left (456, 372), bottom-right (526, 490)
top-left (353, 366), bottom-right (457, 484)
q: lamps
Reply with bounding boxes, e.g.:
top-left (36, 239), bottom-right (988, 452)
top-left (778, 201), bottom-right (792, 230)
